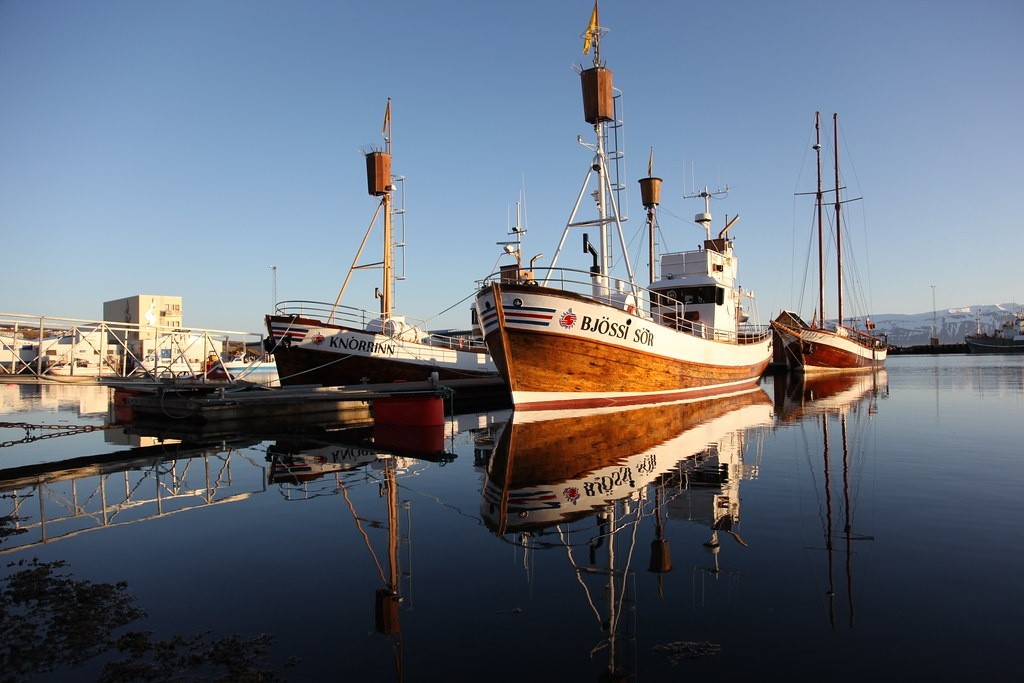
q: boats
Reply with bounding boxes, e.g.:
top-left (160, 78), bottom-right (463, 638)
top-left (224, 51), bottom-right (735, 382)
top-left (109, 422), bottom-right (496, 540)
top-left (964, 308), bottom-right (1024, 354)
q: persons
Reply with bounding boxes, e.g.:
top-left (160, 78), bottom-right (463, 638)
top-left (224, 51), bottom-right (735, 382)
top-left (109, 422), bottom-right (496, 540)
top-left (207, 350), bottom-right (234, 379)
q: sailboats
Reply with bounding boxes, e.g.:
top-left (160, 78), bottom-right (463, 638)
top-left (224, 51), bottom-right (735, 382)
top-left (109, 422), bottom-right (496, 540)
top-left (771, 365), bottom-right (889, 632)
top-left (471, 0), bottom-right (776, 426)
top-left (265, 97), bottom-right (514, 414)
top-left (263, 411), bottom-right (482, 665)
top-left (769, 110), bottom-right (888, 371)
top-left (479, 384), bottom-right (775, 683)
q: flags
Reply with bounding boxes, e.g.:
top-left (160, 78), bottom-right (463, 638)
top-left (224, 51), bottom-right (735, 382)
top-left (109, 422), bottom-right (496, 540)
top-left (382, 105), bottom-right (389, 133)
top-left (583, 6), bottom-right (597, 54)
